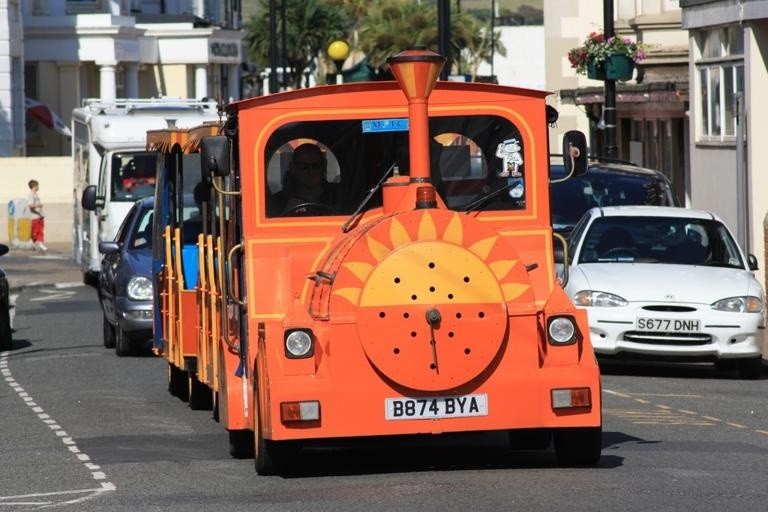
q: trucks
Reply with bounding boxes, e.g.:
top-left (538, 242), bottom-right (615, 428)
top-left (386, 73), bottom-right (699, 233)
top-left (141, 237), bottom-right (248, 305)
top-left (70, 96), bottom-right (228, 286)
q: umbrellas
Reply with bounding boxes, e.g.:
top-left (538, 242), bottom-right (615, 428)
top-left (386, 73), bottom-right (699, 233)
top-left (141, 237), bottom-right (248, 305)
top-left (25, 96), bottom-right (72, 138)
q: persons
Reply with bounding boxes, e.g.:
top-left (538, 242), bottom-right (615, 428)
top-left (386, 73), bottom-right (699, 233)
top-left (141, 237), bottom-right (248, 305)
top-left (27, 179), bottom-right (48, 252)
top-left (120, 160), bottom-right (156, 193)
top-left (182, 181), bottom-right (229, 243)
top-left (270, 143), bottom-right (344, 217)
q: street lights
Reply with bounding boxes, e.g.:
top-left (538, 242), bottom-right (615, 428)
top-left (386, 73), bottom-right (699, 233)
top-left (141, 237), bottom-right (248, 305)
top-left (327, 41), bottom-right (349, 85)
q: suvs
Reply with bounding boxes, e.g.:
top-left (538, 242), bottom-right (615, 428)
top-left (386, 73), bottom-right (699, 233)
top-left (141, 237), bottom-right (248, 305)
top-left (549, 163), bottom-right (681, 278)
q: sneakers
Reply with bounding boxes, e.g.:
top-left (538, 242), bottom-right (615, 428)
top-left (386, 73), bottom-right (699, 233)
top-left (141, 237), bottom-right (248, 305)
top-left (33, 241), bottom-right (46, 251)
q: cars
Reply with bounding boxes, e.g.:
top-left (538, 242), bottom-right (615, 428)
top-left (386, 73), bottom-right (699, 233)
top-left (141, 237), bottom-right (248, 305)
top-left (96, 193), bottom-right (230, 357)
top-left (556, 205), bottom-right (765, 379)
top-left (0, 243), bottom-right (13, 351)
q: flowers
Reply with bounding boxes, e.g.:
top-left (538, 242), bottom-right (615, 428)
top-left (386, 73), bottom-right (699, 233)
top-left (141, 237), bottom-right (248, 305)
top-left (568, 32), bottom-right (654, 76)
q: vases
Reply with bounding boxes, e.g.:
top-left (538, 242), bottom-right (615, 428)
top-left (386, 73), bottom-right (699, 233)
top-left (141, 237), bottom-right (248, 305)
top-left (587, 54), bottom-right (633, 81)
